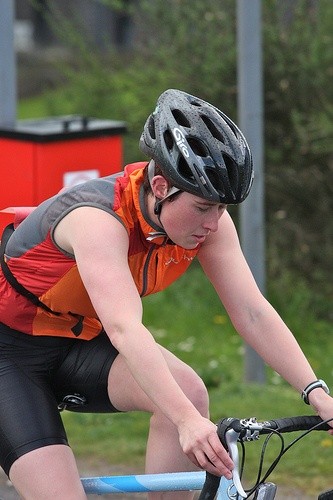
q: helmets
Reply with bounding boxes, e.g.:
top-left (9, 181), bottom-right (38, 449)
top-left (140, 90), bottom-right (254, 206)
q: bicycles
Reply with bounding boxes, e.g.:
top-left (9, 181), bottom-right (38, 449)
top-left (80, 415), bottom-right (332, 500)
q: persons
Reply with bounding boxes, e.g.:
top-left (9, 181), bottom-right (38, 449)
top-left (0, 88), bottom-right (332, 500)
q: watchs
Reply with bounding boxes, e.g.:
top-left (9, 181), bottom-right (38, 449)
top-left (300, 379), bottom-right (330, 405)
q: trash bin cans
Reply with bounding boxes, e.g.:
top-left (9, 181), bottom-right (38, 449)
top-left (0, 114), bottom-right (129, 256)
